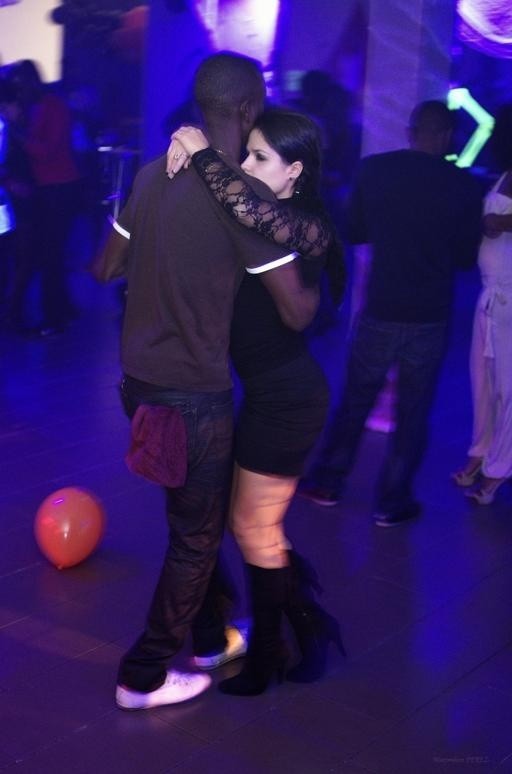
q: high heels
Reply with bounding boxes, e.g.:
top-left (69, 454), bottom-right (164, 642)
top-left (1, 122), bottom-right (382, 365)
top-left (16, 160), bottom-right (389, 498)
top-left (467, 471), bottom-right (511, 506)
top-left (450, 457), bottom-right (488, 491)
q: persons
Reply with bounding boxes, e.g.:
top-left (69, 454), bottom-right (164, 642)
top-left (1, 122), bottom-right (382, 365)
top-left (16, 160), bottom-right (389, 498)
top-left (292, 68), bottom-right (358, 302)
top-left (93, 51), bottom-right (322, 710)
top-left (1, 75), bottom-right (27, 330)
top-left (449, 101), bottom-right (512, 508)
top-left (163, 104), bottom-right (350, 701)
top-left (10, 58), bottom-right (77, 332)
top-left (294, 101), bottom-right (482, 529)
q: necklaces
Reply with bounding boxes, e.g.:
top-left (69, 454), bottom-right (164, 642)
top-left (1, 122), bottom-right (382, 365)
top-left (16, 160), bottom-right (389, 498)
top-left (211, 147), bottom-right (229, 156)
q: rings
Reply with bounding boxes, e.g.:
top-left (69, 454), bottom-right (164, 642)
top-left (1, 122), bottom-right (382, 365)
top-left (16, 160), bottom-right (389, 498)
top-left (181, 152), bottom-right (189, 159)
top-left (174, 153), bottom-right (180, 160)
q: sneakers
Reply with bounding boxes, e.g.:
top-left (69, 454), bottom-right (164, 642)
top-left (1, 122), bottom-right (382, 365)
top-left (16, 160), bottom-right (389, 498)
top-left (192, 617), bottom-right (257, 671)
top-left (372, 498), bottom-right (423, 531)
top-left (297, 476), bottom-right (341, 509)
top-left (113, 668), bottom-right (215, 713)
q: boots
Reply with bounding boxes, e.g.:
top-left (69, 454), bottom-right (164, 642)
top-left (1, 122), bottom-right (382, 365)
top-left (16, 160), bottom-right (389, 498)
top-left (219, 548), bottom-right (352, 699)
